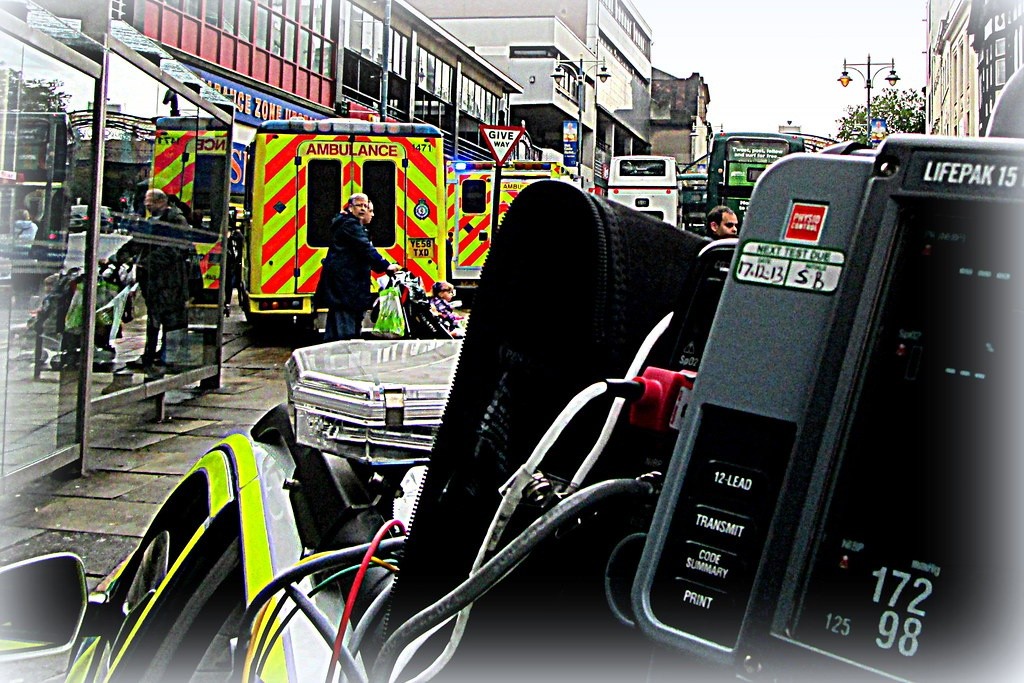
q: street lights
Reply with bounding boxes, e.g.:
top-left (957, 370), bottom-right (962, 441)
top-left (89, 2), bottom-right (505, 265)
top-left (837, 52), bottom-right (902, 148)
top-left (549, 52), bottom-right (612, 192)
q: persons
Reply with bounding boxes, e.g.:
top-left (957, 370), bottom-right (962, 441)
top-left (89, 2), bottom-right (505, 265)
top-left (314, 192), bottom-right (467, 344)
top-left (11, 184), bottom-right (205, 370)
top-left (706, 206), bottom-right (739, 238)
top-left (223, 220), bottom-right (245, 317)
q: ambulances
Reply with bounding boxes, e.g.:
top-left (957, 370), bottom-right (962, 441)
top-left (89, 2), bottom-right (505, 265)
top-left (446, 159), bottom-right (588, 290)
top-left (143, 115), bottom-right (251, 302)
top-left (241, 116), bottom-right (447, 336)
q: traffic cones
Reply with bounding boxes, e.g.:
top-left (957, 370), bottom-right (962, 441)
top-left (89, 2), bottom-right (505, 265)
top-left (29, 203), bottom-right (126, 262)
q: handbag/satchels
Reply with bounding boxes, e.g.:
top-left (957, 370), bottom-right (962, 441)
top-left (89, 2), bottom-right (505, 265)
top-left (63, 280), bottom-right (119, 336)
top-left (371, 287), bottom-right (407, 337)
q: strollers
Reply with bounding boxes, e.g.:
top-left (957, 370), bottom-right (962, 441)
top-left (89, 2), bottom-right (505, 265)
top-left (54, 256), bottom-right (140, 372)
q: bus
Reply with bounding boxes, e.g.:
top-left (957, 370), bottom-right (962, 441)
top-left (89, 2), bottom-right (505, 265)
top-left (606, 155), bottom-right (686, 231)
top-left (705, 131), bottom-right (806, 234)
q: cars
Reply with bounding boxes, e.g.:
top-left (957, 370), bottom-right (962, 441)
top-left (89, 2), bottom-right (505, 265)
top-left (1, 332), bottom-right (486, 683)
